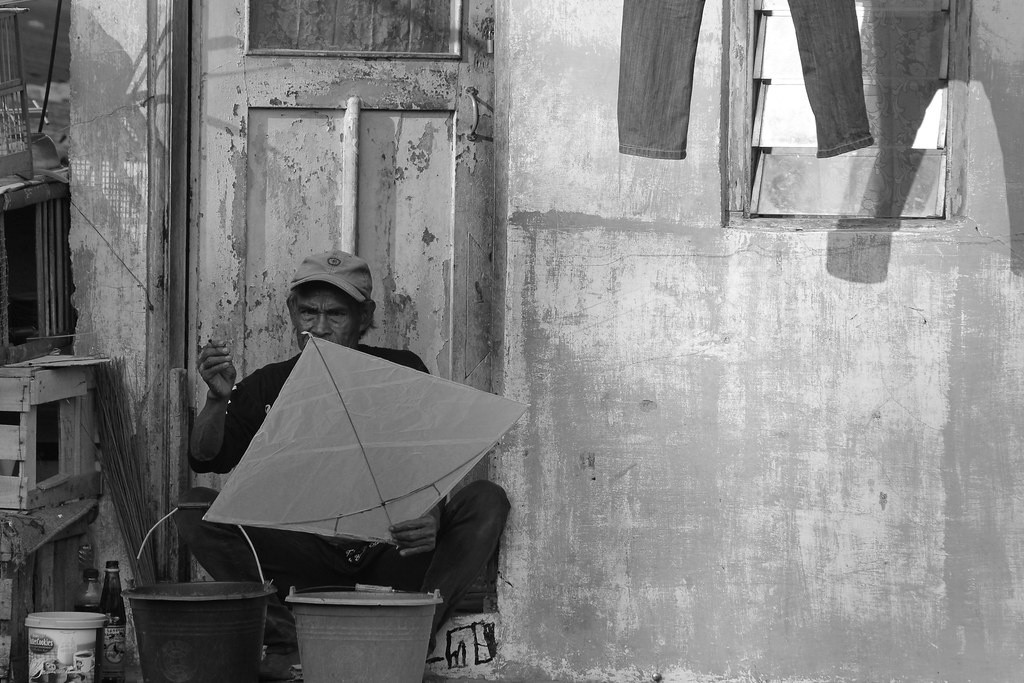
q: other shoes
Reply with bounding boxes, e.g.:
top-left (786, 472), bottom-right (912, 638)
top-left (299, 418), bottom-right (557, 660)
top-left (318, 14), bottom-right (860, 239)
top-left (258, 642), bottom-right (300, 681)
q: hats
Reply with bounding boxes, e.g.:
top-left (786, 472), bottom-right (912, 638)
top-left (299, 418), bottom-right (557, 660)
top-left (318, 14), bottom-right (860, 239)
top-left (290, 251), bottom-right (373, 302)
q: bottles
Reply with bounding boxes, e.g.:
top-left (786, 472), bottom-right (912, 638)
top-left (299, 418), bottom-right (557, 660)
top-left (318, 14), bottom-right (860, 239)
top-left (96, 561), bottom-right (126, 683)
top-left (57, 630), bottom-right (77, 665)
top-left (73, 569), bottom-right (103, 613)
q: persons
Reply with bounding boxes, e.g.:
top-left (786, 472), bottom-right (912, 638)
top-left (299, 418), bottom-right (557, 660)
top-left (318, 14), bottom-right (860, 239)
top-left (187, 251), bottom-right (512, 683)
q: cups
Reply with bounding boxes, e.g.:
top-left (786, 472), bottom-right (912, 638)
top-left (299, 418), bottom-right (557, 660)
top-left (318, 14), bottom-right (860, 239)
top-left (73, 650), bottom-right (95, 672)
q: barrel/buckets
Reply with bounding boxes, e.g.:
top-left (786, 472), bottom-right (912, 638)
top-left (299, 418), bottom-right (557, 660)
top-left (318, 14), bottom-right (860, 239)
top-left (25, 611), bottom-right (108, 683)
top-left (286, 583), bottom-right (444, 683)
top-left (120, 502), bottom-right (279, 682)
